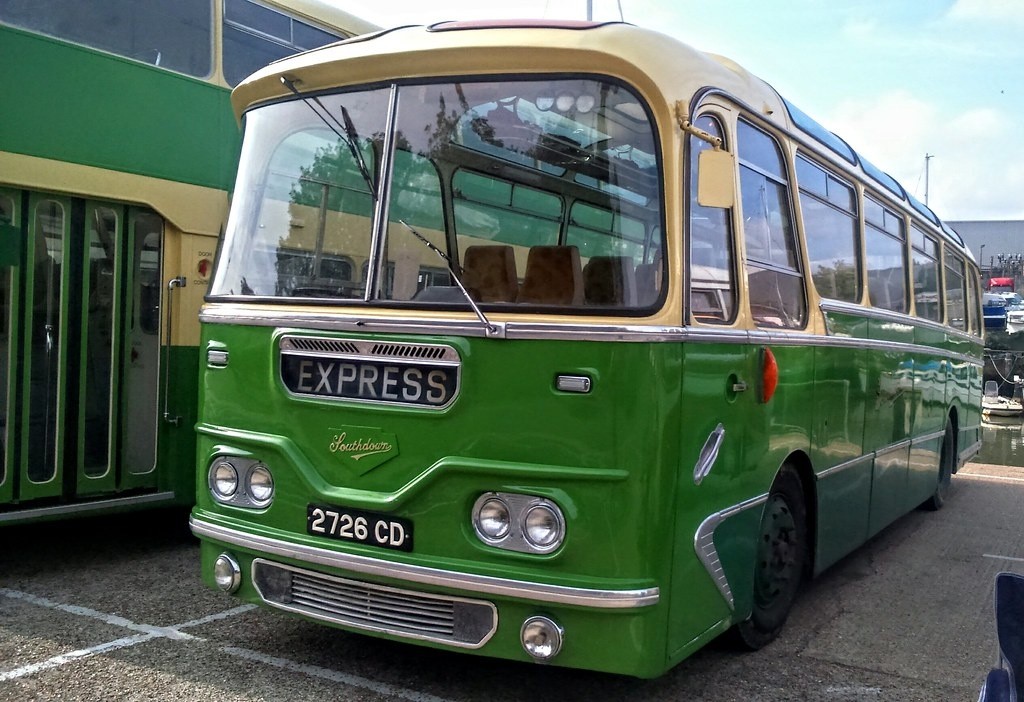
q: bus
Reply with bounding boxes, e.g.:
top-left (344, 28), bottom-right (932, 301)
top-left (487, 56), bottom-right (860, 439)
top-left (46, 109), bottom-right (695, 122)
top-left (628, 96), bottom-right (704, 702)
top-left (189, 21), bottom-right (986, 677)
top-left (0, 0), bottom-right (714, 526)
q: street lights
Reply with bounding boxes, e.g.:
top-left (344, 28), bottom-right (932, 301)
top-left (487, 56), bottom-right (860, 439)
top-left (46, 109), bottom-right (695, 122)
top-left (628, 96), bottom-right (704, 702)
top-left (979, 244), bottom-right (985, 275)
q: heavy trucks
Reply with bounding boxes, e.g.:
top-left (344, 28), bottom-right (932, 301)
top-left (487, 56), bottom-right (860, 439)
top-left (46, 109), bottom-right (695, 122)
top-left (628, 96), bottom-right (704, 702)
top-left (990, 278), bottom-right (1015, 293)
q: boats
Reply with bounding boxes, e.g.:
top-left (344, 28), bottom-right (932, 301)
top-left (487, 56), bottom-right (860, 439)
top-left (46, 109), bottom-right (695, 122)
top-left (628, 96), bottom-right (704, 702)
top-left (982, 381), bottom-right (1023, 417)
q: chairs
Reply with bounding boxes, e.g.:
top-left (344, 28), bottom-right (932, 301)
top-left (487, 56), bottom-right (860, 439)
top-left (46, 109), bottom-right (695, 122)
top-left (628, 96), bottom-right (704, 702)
top-left (458, 244), bottom-right (799, 319)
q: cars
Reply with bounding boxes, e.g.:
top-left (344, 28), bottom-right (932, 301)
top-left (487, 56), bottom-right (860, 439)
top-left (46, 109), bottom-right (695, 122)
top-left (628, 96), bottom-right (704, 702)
top-left (984, 291), bottom-right (1024, 324)
